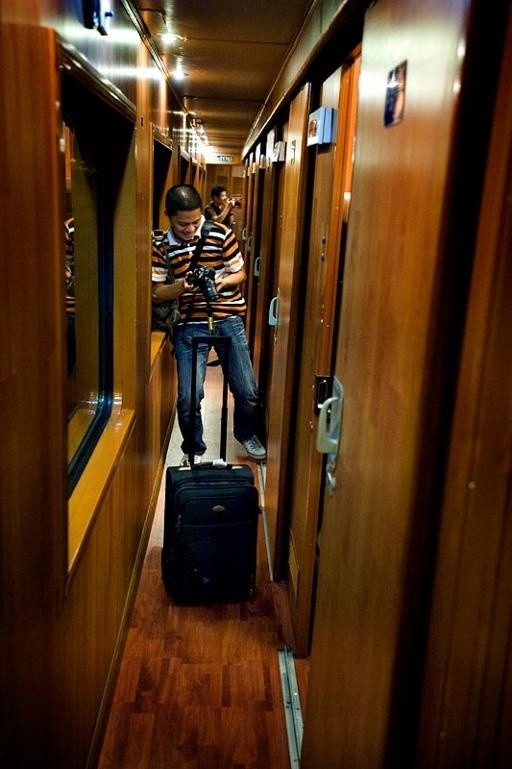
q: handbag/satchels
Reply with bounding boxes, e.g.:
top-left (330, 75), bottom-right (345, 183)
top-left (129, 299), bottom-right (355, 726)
top-left (157, 300), bottom-right (179, 331)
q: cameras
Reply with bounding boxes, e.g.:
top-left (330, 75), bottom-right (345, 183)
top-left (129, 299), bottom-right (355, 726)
top-left (185, 266), bottom-right (219, 303)
top-left (230, 198), bottom-right (241, 207)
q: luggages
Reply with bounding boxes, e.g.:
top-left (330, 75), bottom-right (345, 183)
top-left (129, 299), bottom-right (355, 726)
top-left (161, 464), bottom-right (257, 606)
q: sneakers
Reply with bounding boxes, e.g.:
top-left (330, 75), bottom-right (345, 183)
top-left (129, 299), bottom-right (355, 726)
top-left (180, 452), bottom-right (203, 465)
top-left (232, 429), bottom-right (267, 460)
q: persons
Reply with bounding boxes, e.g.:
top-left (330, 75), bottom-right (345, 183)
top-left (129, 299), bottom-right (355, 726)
top-left (202, 186), bottom-right (235, 229)
top-left (149, 185), bottom-right (268, 466)
